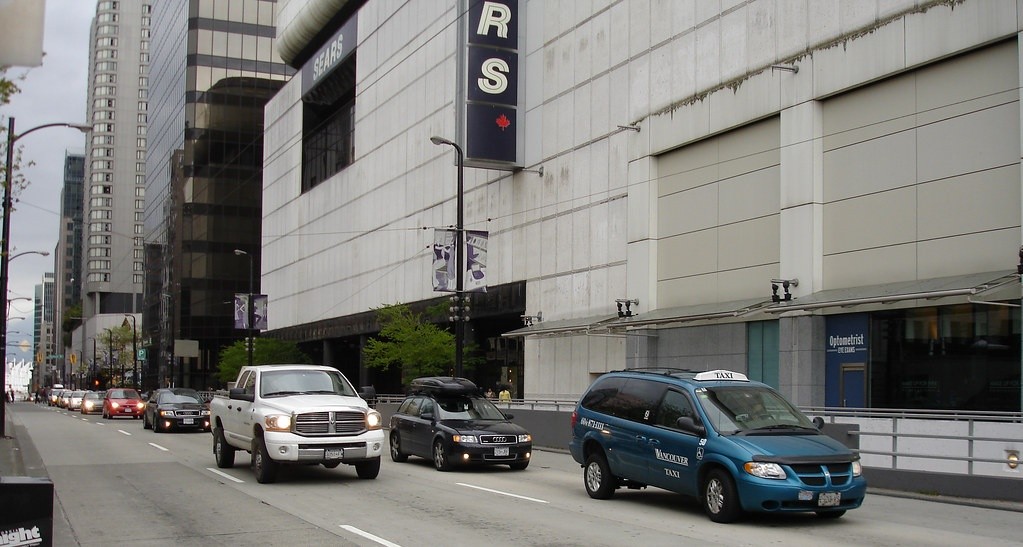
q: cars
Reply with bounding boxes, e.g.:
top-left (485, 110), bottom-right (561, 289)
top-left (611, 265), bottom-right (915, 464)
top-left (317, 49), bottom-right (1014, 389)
top-left (67, 391), bottom-right (90, 411)
top-left (102, 389), bottom-right (145, 420)
top-left (389, 394), bottom-right (532, 471)
top-left (80, 392), bottom-right (106, 414)
top-left (142, 388), bottom-right (211, 433)
top-left (48, 389), bottom-right (73, 409)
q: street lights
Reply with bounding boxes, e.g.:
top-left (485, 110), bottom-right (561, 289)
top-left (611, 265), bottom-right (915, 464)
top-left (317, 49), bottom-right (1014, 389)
top-left (103, 328), bottom-right (113, 388)
top-left (76, 349), bottom-right (83, 390)
top-left (88, 337), bottom-right (96, 391)
top-left (0, 116), bottom-right (93, 437)
top-left (164, 294), bottom-right (174, 388)
top-left (431, 136), bottom-right (464, 378)
top-left (125, 314), bottom-right (137, 388)
top-left (234, 249), bottom-right (253, 365)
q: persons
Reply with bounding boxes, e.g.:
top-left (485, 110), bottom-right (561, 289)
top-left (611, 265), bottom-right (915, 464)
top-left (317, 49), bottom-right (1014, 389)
top-left (35, 389), bottom-right (48, 404)
top-left (499, 386), bottom-right (512, 402)
top-left (487, 388), bottom-right (493, 398)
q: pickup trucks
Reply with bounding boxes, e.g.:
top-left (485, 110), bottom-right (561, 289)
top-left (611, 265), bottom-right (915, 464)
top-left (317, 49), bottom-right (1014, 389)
top-left (209, 364), bottom-right (385, 484)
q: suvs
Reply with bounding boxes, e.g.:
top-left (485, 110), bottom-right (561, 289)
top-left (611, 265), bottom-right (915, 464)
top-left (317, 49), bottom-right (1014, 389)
top-left (568, 367), bottom-right (866, 522)
